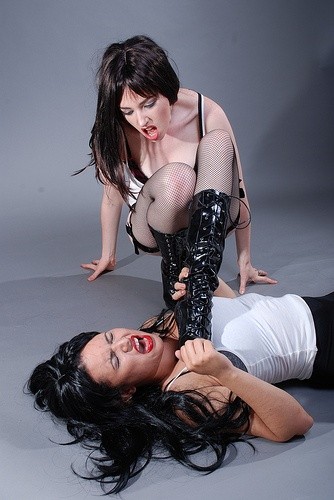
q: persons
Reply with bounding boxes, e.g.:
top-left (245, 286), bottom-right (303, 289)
top-left (22, 267), bottom-right (334, 496)
top-left (70, 35), bottom-right (277, 350)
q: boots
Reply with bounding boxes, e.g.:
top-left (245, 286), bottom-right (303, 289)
top-left (148, 223), bottom-right (189, 310)
top-left (173, 188), bottom-right (231, 339)
